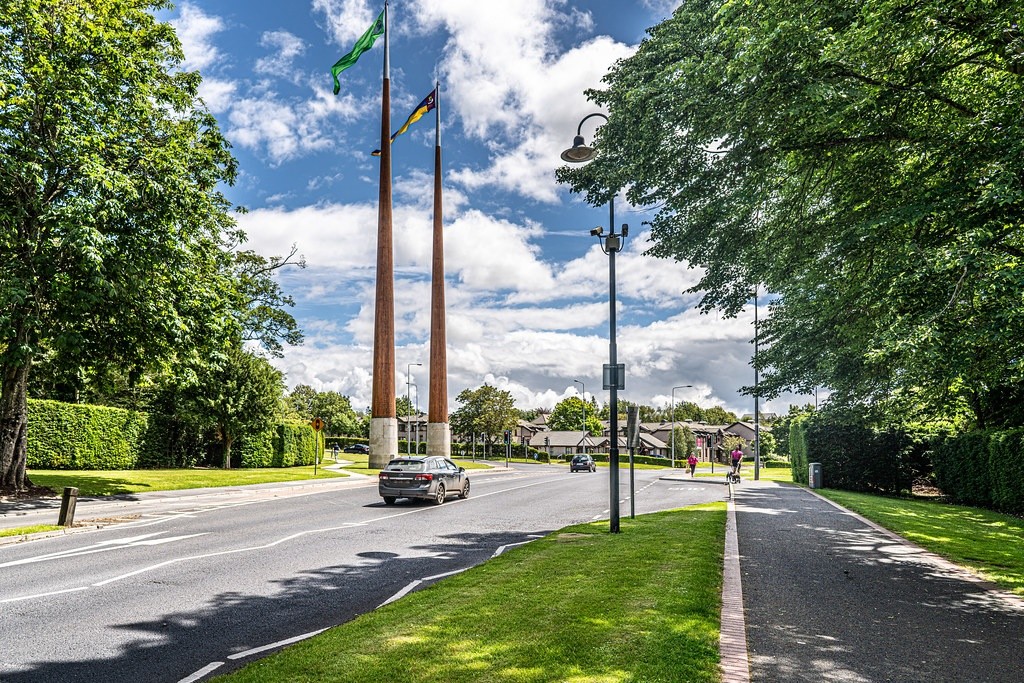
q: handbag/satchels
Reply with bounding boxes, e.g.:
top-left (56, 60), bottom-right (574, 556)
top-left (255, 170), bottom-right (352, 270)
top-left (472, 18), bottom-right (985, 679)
top-left (685, 468), bottom-right (690, 473)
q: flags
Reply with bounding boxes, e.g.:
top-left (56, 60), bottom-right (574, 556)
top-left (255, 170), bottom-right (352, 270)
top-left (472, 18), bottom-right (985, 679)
top-left (331, 12), bottom-right (384, 94)
top-left (373, 89), bottom-right (436, 155)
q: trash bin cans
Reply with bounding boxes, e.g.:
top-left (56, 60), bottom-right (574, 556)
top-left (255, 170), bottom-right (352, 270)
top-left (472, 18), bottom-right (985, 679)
top-left (808, 462), bottom-right (823, 489)
top-left (566, 455), bottom-right (574, 462)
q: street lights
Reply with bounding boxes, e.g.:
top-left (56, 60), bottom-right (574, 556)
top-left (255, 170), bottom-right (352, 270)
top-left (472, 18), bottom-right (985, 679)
top-left (408, 363), bottom-right (422, 454)
top-left (574, 379), bottom-right (585, 453)
top-left (406, 382), bottom-right (418, 455)
top-left (672, 385), bottom-right (692, 468)
top-left (561, 113), bottom-right (618, 535)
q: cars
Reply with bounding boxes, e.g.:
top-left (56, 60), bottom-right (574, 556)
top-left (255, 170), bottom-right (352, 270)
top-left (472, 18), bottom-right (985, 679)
top-left (344, 445), bottom-right (369, 455)
top-left (570, 454), bottom-right (597, 472)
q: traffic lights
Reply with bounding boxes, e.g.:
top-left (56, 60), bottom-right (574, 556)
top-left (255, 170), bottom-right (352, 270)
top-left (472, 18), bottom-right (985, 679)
top-left (545, 437), bottom-right (548, 444)
top-left (504, 430), bottom-right (509, 444)
top-left (521, 437), bottom-right (524, 445)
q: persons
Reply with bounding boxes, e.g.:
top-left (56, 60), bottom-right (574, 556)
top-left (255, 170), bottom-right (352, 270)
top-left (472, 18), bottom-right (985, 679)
top-left (333, 442), bottom-right (341, 460)
top-left (687, 452), bottom-right (699, 478)
top-left (731, 446), bottom-right (743, 472)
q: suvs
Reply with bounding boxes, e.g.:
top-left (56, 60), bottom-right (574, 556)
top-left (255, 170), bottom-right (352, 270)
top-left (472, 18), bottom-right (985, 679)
top-left (378, 456), bottom-right (470, 505)
